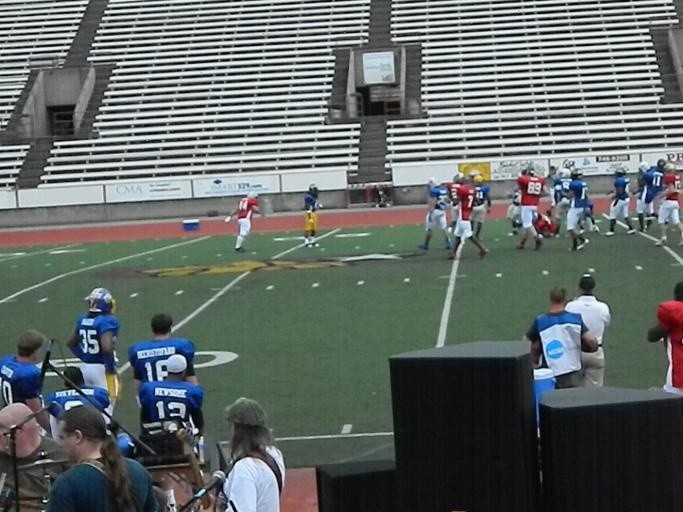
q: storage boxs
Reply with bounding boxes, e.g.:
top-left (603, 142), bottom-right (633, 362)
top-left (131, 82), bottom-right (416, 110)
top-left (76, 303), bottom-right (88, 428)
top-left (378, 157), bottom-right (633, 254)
top-left (182, 219), bottom-right (200, 231)
top-left (533, 366), bottom-right (556, 426)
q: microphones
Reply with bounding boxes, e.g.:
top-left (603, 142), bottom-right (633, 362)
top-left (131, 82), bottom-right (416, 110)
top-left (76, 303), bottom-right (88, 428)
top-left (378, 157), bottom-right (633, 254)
top-left (191, 468), bottom-right (226, 499)
top-left (3, 399), bottom-right (57, 436)
top-left (39, 337), bottom-right (54, 386)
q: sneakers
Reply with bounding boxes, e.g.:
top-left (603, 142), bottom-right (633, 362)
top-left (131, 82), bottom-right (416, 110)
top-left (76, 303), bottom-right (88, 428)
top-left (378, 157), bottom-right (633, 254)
top-left (626, 224), bottom-right (649, 235)
top-left (234, 247), bottom-right (246, 252)
top-left (568, 237), bottom-right (589, 252)
top-left (604, 231), bottom-right (615, 235)
top-left (509, 230), bottom-right (544, 251)
top-left (418, 244), bottom-right (429, 250)
top-left (655, 237), bottom-right (668, 246)
top-left (479, 248), bottom-right (489, 260)
top-left (445, 246), bottom-right (456, 260)
top-left (306, 242), bottom-right (320, 248)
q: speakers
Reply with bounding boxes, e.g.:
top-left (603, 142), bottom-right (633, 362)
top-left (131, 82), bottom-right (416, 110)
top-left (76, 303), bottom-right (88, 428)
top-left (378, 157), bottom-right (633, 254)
top-left (539, 384), bottom-right (683, 511)
top-left (387, 339), bottom-right (542, 511)
top-left (315, 457), bottom-right (397, 512)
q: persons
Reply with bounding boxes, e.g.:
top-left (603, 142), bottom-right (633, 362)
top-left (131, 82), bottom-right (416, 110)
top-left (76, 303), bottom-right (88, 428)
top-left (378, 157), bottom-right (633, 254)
top-left (127, 313), bottom-right (198, 411)
top-left (225, 190), bottom-right (264, 250)
top-left (417, 154), bottom-right (683, 259)
top-left (304, 182), bottom-right (324, 248)
top-left (219, 397), bottom-right (286, 512)
top-left (563, 273), bottom-right (611, 388)
top-left (647, 281), bottom-right (683, 398)
top-left (65, 286), bottom-right (123, 425)
top-left (1, 329), bottom-right (205, 512)
top-left (520, 283), bottom-right (599, 389)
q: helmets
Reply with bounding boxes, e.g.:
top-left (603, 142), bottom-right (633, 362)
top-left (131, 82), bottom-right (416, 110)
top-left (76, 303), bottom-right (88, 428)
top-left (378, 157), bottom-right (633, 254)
top-left (247, 191), bottom-right (258, 199)
top-left (116, 432), bottom-right (136, 459)
top-left (428, 170), bottom-right (485, 188)
top-left (615, 168), bottom-right (626, 174)
top-left (639, 159), bottom-right (674, 173)
top-left (309, 183), bottom-right (316, 190)
top-left (84, 288), bottom-right (116, 314)
top-left (522, 166), bottom-right (583, 180)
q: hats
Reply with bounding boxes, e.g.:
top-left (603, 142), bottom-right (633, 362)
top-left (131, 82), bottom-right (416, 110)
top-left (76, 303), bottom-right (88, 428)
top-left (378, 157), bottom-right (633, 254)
top-left (225, 397), bottom-right (267, 427)
top-left (166, 353), bottom-right (188, 373)
